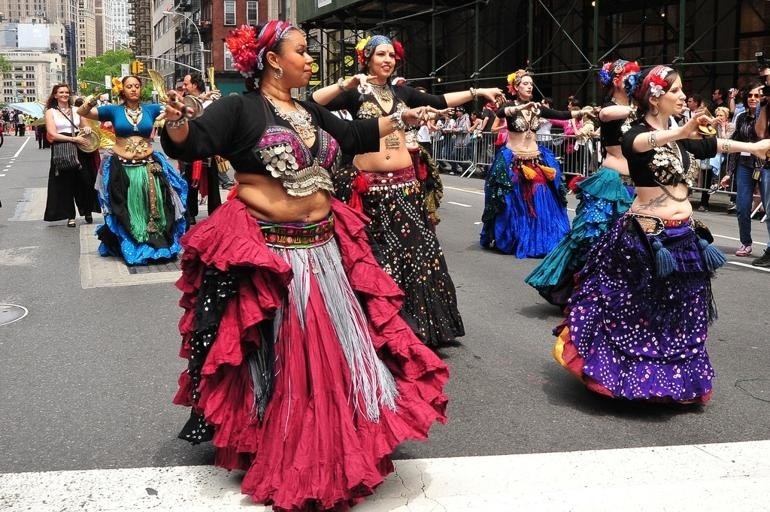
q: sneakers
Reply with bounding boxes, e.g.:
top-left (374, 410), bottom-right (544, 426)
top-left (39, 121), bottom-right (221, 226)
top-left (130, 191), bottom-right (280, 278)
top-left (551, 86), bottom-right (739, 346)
top-left (752, 247), bottom-right (769, 267)
top-left (735, 245), bottom-right (752, 256)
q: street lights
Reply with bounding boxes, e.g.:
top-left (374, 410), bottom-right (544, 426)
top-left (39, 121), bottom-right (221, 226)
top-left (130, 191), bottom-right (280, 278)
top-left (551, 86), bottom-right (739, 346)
top-left (161, 9), bottom-right (206, 94)
top-left (95, 59), bottom-right (115, 106)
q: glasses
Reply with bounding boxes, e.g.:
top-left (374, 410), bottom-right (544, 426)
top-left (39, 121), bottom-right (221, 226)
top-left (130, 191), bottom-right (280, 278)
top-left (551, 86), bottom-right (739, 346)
top-left (747, 93), bottom-right (759, 98)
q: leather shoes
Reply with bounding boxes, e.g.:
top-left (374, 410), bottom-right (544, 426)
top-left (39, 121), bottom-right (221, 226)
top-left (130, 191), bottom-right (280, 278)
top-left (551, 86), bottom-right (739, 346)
top-left (67, 219), bottom-right (76, 227)
top-left (85, 211), bottom-right (92, 223)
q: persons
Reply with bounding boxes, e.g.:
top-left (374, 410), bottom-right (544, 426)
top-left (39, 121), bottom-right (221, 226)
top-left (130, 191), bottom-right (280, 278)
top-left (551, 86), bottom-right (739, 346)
top-left (555, 65), bottom-right (770, 404)
top-left (0, 21), bottom-right (770, 512)
top-left (479, 70), bottom-right (600, 259)
top-left (527, 60), bottom-right (641, 311)
top-left (310, 32), bottom-right (507, 345)
top-left (417, 94), bottom-right (505, 174)
top-left (1, 73), bottom-right (223, 266)
top-left (682, 65), bottom-right (770, 266)
top-left (157, 20), bottom-right (452, 511)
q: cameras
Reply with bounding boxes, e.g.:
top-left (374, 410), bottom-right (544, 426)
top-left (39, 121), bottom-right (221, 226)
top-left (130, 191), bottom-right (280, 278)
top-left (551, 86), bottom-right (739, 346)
top-left (729, 90), bottom-right (732, 94)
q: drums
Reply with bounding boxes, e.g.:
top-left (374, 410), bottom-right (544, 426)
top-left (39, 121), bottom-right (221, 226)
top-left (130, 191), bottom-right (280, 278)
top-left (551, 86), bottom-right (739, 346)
top-left (76, 126), bottom-right (101, 152)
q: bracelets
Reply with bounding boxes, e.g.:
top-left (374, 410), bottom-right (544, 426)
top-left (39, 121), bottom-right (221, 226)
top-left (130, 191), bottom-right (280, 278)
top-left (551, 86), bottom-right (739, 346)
top-left (648, 131), bottom-right (659, 149)
top-left (469, 87), bottom-right (478, 102)
top-left (391, 109), bottom-right (413, 131)
top-left (338, 78), bottom-right (350, 92)
top-left (630, 106), bottom-right (637, 118)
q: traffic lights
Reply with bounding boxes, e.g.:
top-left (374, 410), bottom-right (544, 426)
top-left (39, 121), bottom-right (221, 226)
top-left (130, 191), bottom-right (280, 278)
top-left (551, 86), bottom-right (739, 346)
top-left (85, 84), bottom-right (87, 88)
top-left (81, 83), bottom-right (84, 88)
top-left (138, 61), bottom-right (144, 75)
